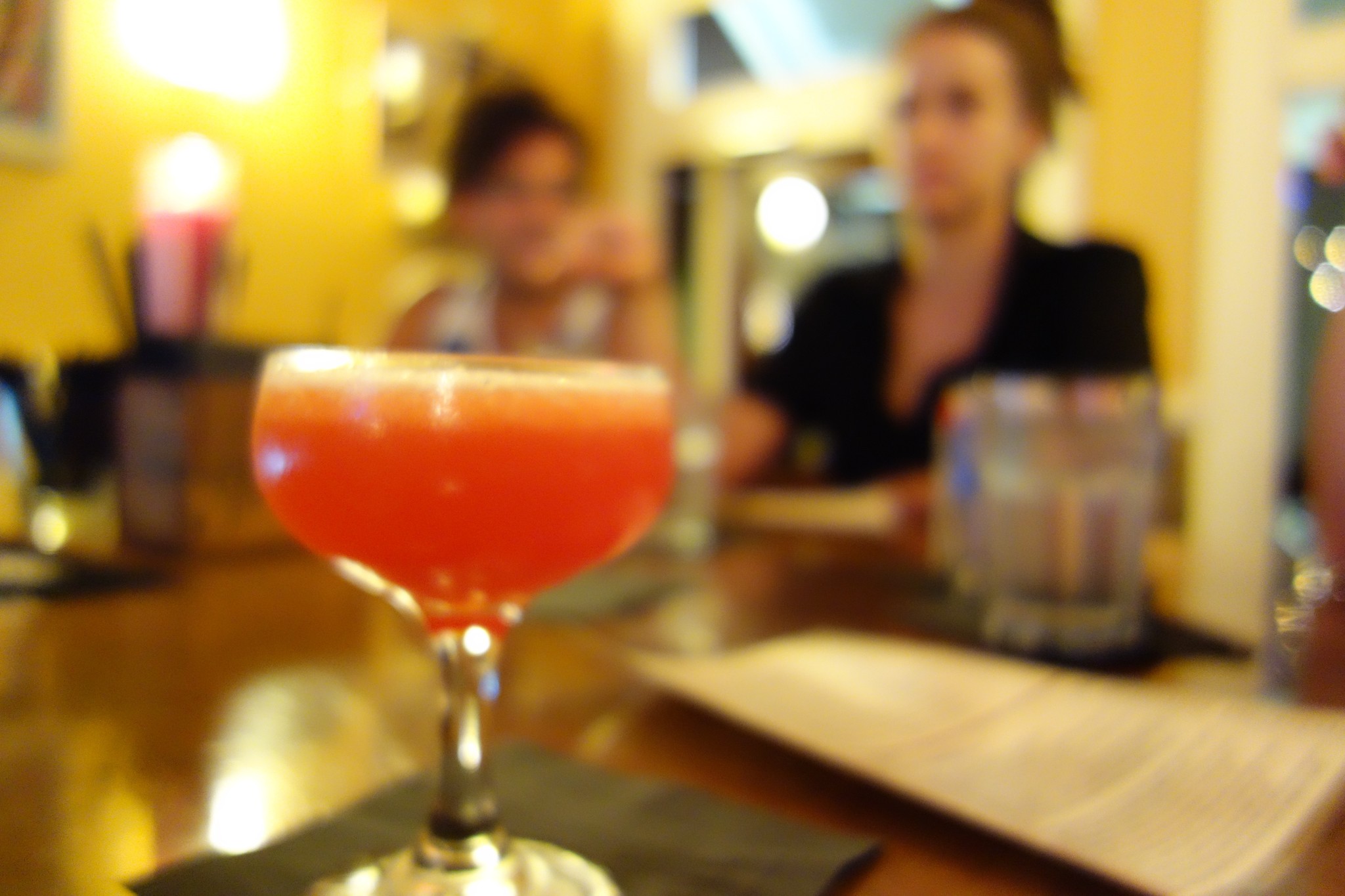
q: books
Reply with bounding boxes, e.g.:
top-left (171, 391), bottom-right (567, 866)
top-left (565, 620), bottom-right (1345, 896)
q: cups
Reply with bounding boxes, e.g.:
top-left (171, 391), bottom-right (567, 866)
top-left (933, 382), bottom-right (1163, 656)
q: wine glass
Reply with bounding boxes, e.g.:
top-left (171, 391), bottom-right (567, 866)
top-left (223, 340), bottom-right (678, 894)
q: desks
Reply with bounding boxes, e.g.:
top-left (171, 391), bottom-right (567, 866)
top-left (0, 514), bottom-right (1233, 896)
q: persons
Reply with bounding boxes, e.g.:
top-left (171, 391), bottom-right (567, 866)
top-left (711, 0), bottom-right (1164, 526)
top-left (381, 83), bottom-right (675, 395)
